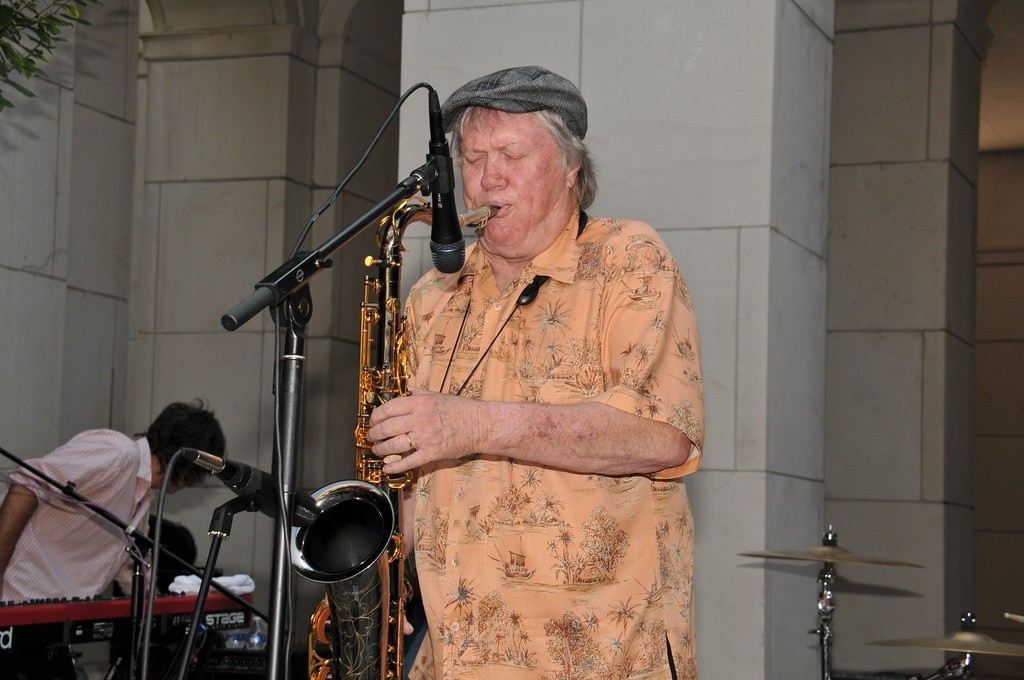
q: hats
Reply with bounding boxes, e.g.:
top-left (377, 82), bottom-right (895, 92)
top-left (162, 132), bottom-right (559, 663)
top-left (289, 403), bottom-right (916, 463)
top-left (441, 65), bottom-right (588, 140)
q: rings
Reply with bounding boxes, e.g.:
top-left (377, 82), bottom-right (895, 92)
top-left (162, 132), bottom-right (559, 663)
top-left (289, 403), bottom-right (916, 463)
top-left (406, 433), bottom-right (413, 450)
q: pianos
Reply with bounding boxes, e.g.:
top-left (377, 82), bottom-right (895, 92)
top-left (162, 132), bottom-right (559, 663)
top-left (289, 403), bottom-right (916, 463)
top-left (1, 589), bottom-right (255, 626)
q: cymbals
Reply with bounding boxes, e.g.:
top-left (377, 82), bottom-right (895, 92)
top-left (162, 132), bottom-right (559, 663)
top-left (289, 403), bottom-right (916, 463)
top-left (864, 631), bottom-right (1023, 657)
top-left (735, 545), bottom-right (928, 569)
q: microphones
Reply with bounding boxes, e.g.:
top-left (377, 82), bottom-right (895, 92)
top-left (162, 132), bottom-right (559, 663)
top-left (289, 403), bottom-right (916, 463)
top-left (427, 91), bottom-right (465, 273)
top-left (180, 447), bottom-right (322, 528)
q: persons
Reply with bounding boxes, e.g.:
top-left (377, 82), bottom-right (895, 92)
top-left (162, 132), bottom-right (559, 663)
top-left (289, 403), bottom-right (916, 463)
top-left (363, 68), bottom-right (705, 680)
top-left (0, 400), bottom-right (227, 680)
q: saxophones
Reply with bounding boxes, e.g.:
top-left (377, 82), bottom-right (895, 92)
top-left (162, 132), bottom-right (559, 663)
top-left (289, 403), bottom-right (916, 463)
top-left (290, 197), bottom-right (501, 680)
